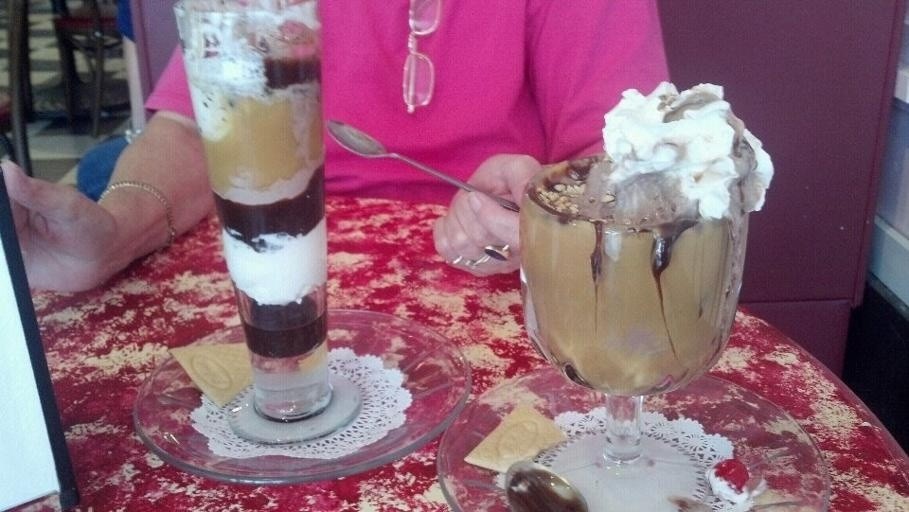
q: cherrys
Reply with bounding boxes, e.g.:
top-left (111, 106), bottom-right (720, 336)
top-left (714, 457), bottom-right (749, 493)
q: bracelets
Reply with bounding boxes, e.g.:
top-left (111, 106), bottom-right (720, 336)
top-left (97, 181), bottom-right (176, 252)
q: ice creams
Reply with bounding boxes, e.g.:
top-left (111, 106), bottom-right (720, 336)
top-left (172, 2), bottom-right (327, 399)
top-left (518, 78), bottom-right (775, 399)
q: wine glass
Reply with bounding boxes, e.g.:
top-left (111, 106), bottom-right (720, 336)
top-left (516, 155), bottom-right (750, 512)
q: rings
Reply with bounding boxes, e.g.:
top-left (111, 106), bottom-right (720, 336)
top-left (444, 256), bottom-right (461, 267)
top-left (484, 243), bottom-right (511, 262)
top-left (465, 253), bottom-right (488, 268)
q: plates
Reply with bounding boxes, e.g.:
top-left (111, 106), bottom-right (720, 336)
top-left (131, 308), bottom-right (475, 488)
top-left (435, 359), bottom-right (832, 512)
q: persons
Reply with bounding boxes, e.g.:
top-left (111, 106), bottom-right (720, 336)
top-left (1, 0), bottom-right (669, 294)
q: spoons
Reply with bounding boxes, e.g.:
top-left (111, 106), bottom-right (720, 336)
top-left (324, 117), bottom-right (521, 212)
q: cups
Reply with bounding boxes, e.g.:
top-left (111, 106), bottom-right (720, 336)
top-left (168, 1), bottom-right (365, 445)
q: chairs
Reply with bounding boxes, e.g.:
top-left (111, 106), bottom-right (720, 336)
top-left (52, 1), bottom-right (136, 138)
top-left (0, 0), bottom-right (34, 182)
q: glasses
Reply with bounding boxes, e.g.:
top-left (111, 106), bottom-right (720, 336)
top-left (402, 0), bottom-right (441, 115)
